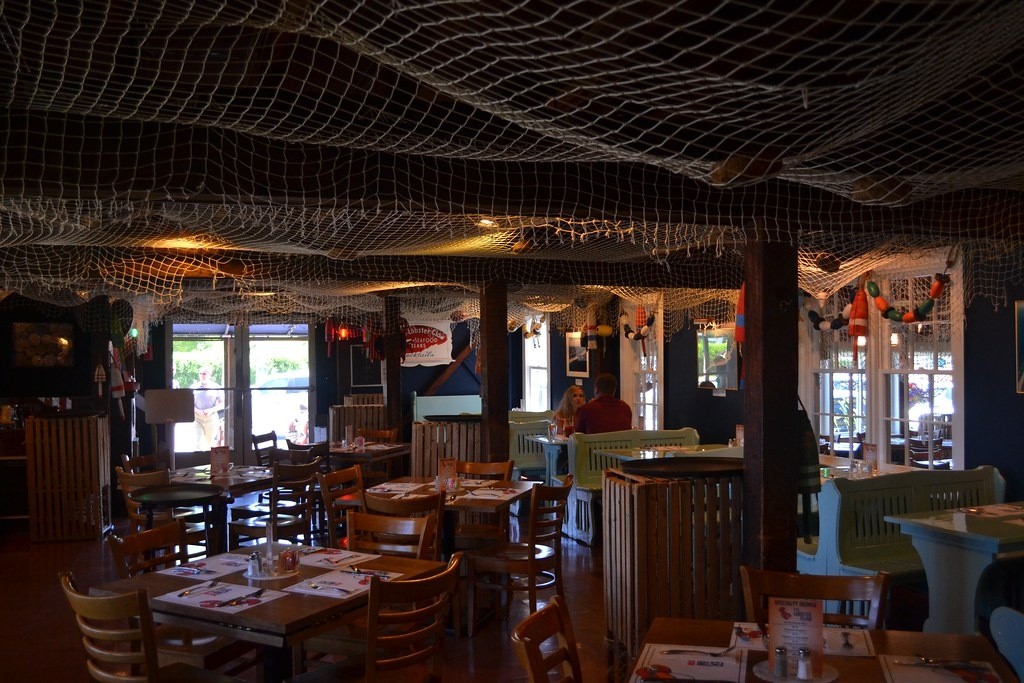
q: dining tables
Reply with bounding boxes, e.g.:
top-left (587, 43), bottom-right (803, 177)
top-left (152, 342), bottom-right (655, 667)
top-left (883, 500), bottom-right (1024, 636)
top-left (329, 440), bottom-right (412, 488)
top-left (334, 476), bottom-right (545, 638)
top-left (525, 434), bottom-right (569, 520)
top-left (87, 539), bottom-right (449, 683)
top-left (819, 454), bottom-right (931, 482)
top-left (626, 615), bottom-right (1021, 683)
top-left (117, 462), bottom-right (273, 557)
top-left (421, 414), bottom-right (481, 426)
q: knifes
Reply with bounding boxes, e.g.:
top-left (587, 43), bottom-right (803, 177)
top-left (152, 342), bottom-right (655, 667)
top-left (211, 588), bottom-right (267, 606)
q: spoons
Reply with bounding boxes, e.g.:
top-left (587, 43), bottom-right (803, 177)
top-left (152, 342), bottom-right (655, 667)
top-left (349, 565), bottom-right (390, 578)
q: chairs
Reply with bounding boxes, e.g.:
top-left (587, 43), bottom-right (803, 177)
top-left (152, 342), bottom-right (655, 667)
top-left (739, 563), bottom-right (890, 632)
top-left (48, 431), bottom-right (582, 683)
top-left (820, 413), bottom-right (953, 462)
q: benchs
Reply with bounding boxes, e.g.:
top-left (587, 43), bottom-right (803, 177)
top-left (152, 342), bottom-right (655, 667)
top-left (411, 391), bottom-right (482, 447)
top-left (796, 462), bottom-right (1007, 627)
top-left (508, 409), bottom-right (702, 550)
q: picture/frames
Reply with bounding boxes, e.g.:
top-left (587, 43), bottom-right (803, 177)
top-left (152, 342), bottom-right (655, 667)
top-left (1014, 300), bottom-right (1024, 393)
top-left (564, 332), bottom-right (590, 379)
top-left (349, 344), bottom-right (383, 386)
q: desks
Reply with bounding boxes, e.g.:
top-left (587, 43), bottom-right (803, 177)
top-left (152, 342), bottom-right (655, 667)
top-left (127, 480), bottom-right (226, 572)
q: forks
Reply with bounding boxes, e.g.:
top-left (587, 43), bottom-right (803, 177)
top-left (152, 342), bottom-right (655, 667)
top-left (178, 576), bottom-right (220, 595)
top-left (661, 645), bottom-right (736, 656)
top-left (307, 579), bottom-right (352, 594)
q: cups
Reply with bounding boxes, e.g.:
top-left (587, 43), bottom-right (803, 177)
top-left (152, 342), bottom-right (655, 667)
top-left (356, 438), bottom-right (365, 452)
top-left (449, 476), bottom-right (454, 488)
top-left (562, 421), bottom-right (574, 441)
top-left (548, 418), bottom-right (556, 440)
top-left (455, 478), bottom-right (461, 488)
top-left (342, 439), bottom-right (347, 449)
top-left (280, 547), bottom-right (298, 571)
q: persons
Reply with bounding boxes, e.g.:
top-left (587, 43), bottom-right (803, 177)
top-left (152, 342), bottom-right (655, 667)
top-left (574, 373), bottom-right (633, 435)
top-left (549, 385), bottom-right (585, 476)
top-left (187, 366), bottom-right (225, 453)
top-left (699, 350), bottom-right (727, 390)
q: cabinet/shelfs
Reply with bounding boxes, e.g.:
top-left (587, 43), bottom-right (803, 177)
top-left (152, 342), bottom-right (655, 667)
top-left (0, 427), bottom-right (31, 520)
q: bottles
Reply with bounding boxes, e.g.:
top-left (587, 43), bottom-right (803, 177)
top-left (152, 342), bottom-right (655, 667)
top-left (253, 551), bottom-right (262, 573)
top-left (849, 459), bottom-right (857, 477)
top-left (729, 438), bottom-right (736, 447)
top-left (775, 646), bottom-right (788, 676)
top-left (434, 475), bottom-right (440, 489)
top-left (798, 647), bottom-right (814, 679)
top-left (866, 450), bottom-right (877, 469)
top-left (248, 555), bottom-right (258, 575)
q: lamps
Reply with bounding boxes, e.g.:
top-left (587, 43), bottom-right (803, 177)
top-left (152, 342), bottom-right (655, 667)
top-left (338, 320), bottom-right (349, 341)
